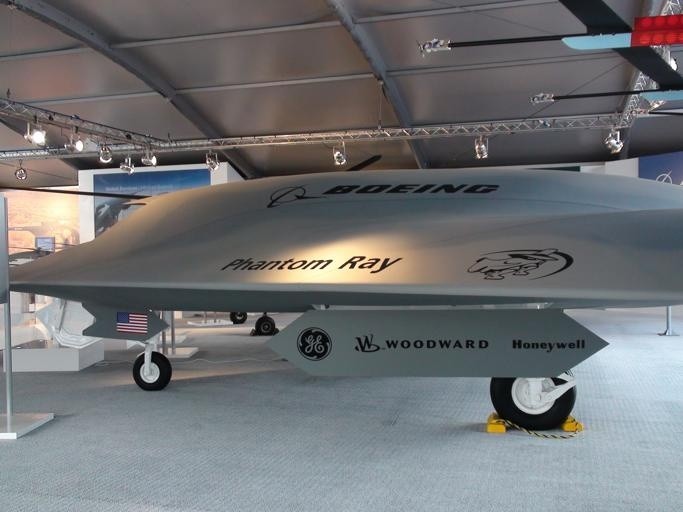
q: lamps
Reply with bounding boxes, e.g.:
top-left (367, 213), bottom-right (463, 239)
top-left (14, 121), bottom-right (157, 181)
top-left (474, 134), bottom-right (489, 159)
top-left (332, 141), bottom-right (346, 165)
top-left (603, 127), bottom-right (624, 154)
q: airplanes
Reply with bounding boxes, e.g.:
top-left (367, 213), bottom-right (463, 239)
top-left (9, 166), bottom-right (683, 431)
